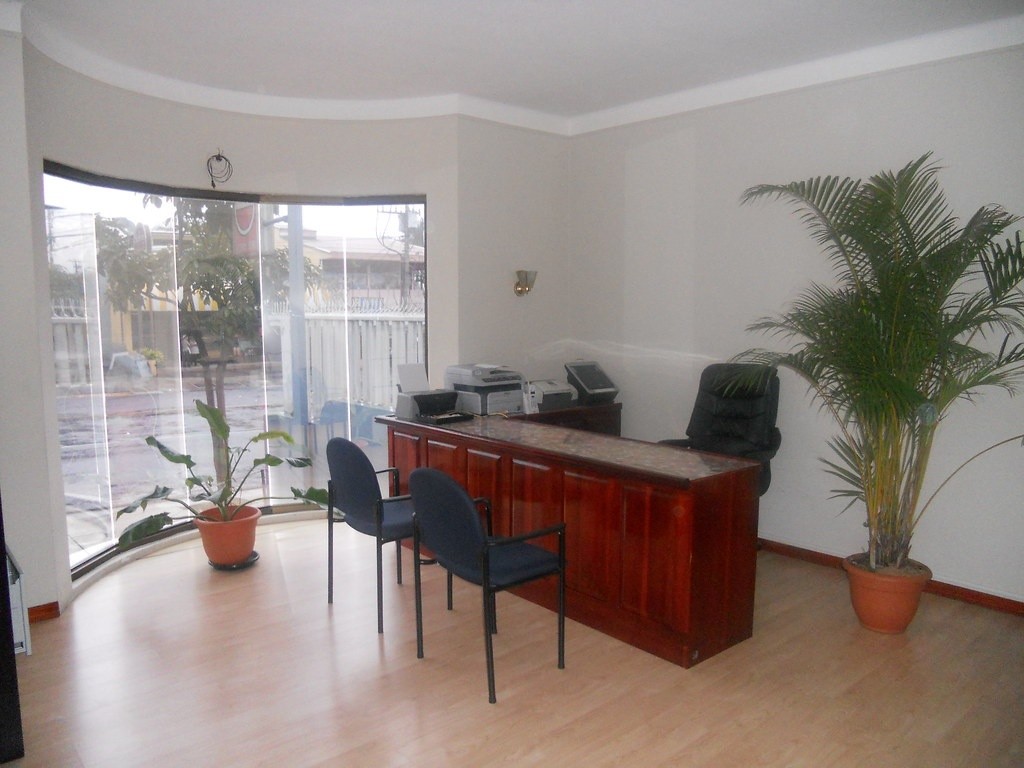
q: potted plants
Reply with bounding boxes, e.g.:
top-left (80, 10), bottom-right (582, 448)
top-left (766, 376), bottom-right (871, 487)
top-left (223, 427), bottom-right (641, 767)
top-left (708, 149), bottom-right (1024, 635)
top-left (115, 399), bottom-right (346, 566)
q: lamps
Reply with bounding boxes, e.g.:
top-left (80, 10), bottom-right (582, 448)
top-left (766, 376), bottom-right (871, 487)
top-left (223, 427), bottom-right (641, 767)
top-left (513, 270), bottom-right (539, 296)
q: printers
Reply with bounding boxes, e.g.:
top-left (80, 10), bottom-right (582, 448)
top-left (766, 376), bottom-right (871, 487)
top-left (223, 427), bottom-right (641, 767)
top-left (444, 362), bottom-right (525, 415)
top-left (394, 385), bottom-right (473, 425)
top-left (526, 378), bottom-right (578, 412)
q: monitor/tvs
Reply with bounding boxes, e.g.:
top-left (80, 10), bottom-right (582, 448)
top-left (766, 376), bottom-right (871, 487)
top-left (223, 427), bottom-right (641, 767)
top-left (564, 359), bottom-right (619, 407)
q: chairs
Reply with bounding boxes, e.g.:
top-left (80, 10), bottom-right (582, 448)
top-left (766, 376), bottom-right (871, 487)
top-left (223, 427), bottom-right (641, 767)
top-left (656, 363), bottom-right (782, 497)
top-left (409, 466), bottom-right (572, 705)
top-left (326, 437), bottom-right (454, 633)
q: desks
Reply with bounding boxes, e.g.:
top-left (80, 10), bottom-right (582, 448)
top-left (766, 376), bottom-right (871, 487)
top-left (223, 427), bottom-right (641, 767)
top-left (372, 403), bottom-right (763, 670)
